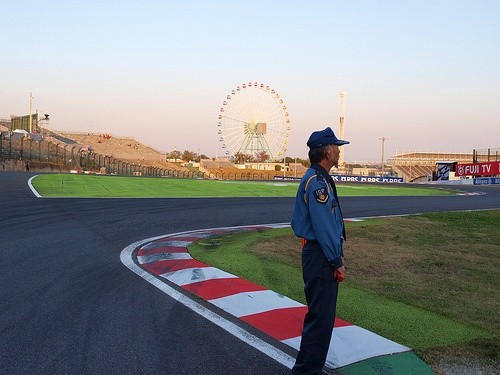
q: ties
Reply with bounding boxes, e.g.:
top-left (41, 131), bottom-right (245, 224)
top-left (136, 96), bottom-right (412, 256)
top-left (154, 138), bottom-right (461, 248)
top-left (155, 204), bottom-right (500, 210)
top-left (329, 176), bottom-right (346, 242)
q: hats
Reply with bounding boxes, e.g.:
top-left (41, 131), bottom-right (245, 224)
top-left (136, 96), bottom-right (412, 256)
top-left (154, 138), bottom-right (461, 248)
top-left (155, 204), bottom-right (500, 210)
top-left (307, 128), bottom-right (350, 149)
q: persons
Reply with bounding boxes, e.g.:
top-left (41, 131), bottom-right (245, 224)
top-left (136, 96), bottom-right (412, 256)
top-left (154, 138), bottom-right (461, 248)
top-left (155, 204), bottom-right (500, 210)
top-left (290, 127), bottom-right (349, 375)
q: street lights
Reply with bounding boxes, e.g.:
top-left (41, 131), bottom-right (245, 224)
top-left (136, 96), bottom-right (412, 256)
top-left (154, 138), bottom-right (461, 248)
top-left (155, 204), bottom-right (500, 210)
top-left (378, 136), bottom-right (389, 177)
